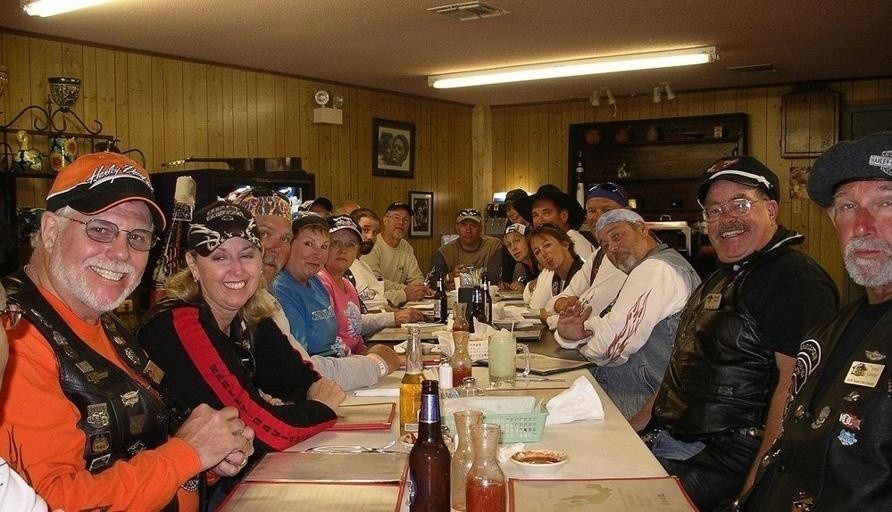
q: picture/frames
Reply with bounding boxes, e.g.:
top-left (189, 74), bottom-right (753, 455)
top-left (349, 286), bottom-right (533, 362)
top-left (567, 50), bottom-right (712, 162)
top-left (409, 192), bottom-right (433, 239)
top-left (373, 118), bottom-right (416, 178)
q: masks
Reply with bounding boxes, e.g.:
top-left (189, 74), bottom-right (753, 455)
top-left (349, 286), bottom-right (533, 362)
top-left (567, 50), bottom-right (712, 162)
top-left (359, 240), bottom-right (374, 255)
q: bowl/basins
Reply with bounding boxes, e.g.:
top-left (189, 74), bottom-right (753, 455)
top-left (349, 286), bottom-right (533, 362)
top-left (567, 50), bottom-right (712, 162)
top-left (510, 449), bottom-right (571, 479)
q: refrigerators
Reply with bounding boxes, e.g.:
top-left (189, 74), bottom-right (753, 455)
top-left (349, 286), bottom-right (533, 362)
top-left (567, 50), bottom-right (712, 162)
top-left (139, 170), bottom-right (316, 322)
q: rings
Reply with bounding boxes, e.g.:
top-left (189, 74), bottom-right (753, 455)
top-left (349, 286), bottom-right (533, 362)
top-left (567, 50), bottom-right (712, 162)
top-left (239, 456), bottom-right (247, 467)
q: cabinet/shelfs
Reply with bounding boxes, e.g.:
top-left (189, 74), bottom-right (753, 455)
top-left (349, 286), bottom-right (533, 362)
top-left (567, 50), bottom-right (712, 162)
top-left (0, 94), bottom-right (146, 286)
top-left (566, 112), bottom-right (749, 260)
top-left (150, 171), bottom-right (315, 223)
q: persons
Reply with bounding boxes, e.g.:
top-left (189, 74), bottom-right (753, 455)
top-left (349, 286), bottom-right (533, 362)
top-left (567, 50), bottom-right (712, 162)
top-left (712, 131), bottom-right (892, 512)
top-left (380, 131), bottom-right (393, 163)
top-left (127, 200), bottom-right (346, 511)
top-left (1, 283), bottom-right (67, 510)
top-left (629, 154), bottom-right (841, 512)
top-left (0, 153), bottom-right (256, 510)
top-left (225, 181), bottom-right (702, 420)
top-left (392, 134), bottom-right (409, 165)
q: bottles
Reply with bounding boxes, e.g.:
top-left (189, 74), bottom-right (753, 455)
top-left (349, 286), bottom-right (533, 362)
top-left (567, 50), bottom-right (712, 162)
top-left (575, 161), bottom-right (585, 183)
top-left (585, 126), bottom-right (659, 145)
top-left (146, 175), bottom-right (199, 309)
top-left (398, 262), bottom-right (532, 512)
top-left (14, 149), bottom-right (44, 174)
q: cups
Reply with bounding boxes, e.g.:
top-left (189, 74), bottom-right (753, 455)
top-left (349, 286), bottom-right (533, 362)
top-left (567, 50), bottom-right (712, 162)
top-left (46, 134), bottom-right (78, 174)
top-left (47, 76), bottom-right (81, 112)
top-left (700, 221), bottom-right (708, 234)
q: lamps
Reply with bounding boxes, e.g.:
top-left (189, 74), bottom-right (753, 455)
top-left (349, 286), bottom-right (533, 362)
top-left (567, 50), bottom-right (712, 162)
top-left (427, 46), bottom-right (719, 89)
top-left (653, 81), bottom-right (677, 103)
top-left (591, 87), bottom-right (615, 108)
top-left (312, 90), bottom-right (344, 126)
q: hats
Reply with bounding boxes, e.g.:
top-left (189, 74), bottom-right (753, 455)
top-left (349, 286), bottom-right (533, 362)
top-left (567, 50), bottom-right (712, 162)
top-left (327, 214), bottom-right (364, 243)
top-left (505, 223), bottom-right (527, 236)
top-left (45, 152), bottom-right (168, 233)
top-left (512, 184), bottom-right (585, 231)
top-left (695, 155), bottom-right (781, 210)
top-left (186, 201), bottom-right (262, 258)
top-left (455, 208), bottom-right (483, 224)
top-left (503, 189), bottom-right (528, 204)
top-left (298, 196), bottom-right (333, 212)
top-left (292, 211), bottom-right (329, 234)
top-left (806, 130), bottom-right (892, 210)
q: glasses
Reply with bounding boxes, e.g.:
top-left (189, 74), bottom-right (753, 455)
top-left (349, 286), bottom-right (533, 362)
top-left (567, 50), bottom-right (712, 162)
top-left (524, 223), bottom-right (558, 237)
top-left (0, 300), bottom-right (26, 331)
top-left (239, 186), bottom-right (290, 205)
top-left (387, 214), bottom-right (409, 224)
top-left (584, 183), bottom-right (628, 207)
top-left (458, 209), bottom-right (482, 219)
top-left (385, 202), bottom-right (415, 217)
top-left (54, 213), bottom-right (158, 251)
top-left (701, 197), bottom-right (768, 223)
